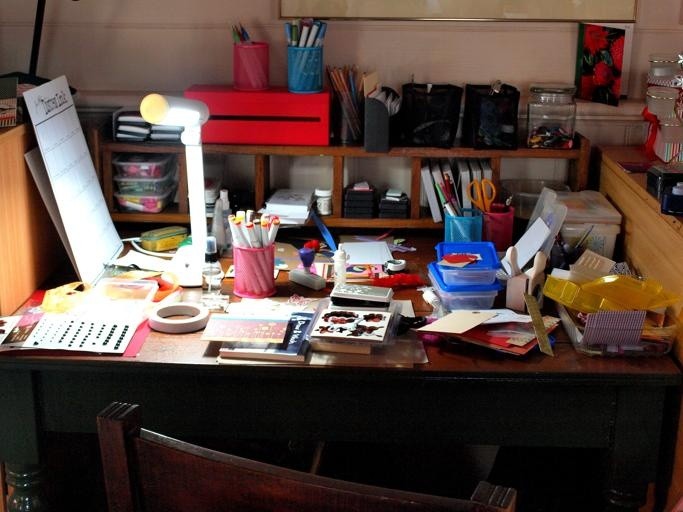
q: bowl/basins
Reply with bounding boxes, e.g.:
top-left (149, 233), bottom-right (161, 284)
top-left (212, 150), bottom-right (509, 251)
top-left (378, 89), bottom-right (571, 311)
top-left (501, 179), bottom-right (571, 218)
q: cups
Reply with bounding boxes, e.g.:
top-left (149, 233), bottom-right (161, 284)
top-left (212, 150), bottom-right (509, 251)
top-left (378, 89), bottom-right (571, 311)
top-left (660, 121), bottom-right (683, 143)
top-left (646, 86), bottom-right (679, 115)
top-left (648, 55), bottom-right (681, 81)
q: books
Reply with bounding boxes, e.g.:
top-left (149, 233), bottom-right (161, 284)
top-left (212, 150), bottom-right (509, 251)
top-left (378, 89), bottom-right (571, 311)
top-left (261, 187), bottom-right (318, 225)
top-left (115, 113), bottom-right (182, 143)
top-left (574, 20), bottom-right (634, 108)
top-left (201, 282), bottom-right (399, 362)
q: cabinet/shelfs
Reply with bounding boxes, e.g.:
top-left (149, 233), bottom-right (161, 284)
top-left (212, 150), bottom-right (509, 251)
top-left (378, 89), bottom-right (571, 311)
top-left (597, 145), bottom-right (683, 511)
top-left (92, 130), bottom-right (590, 227)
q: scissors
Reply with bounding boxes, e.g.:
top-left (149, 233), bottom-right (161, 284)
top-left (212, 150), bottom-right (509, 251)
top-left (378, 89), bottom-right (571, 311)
top-left (395, 311), bottom-right (426, 335)
top-left (467, 178), bottom-right (496, 241)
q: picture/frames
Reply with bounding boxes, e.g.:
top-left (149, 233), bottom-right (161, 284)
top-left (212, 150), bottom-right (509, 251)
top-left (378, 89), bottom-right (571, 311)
top-left (277, 0), bottom-right (640, 23)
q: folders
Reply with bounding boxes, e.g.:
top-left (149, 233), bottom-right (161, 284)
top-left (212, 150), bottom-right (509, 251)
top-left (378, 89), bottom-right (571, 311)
top-left (364, 86), bottom-right (400, 154)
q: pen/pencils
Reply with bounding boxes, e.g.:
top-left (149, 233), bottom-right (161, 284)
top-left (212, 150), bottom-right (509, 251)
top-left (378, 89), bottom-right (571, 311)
top-left (228, 210), bottom-right (279, 294)
top-left (501, 196), bottom-right (512, 213)
top-left (375, 228), bottom-right (393, 241)
top-left (552, 236), bottom-right (571, 257)
top-left (435, 173), bottom-right (468, 243)
top-left (284, 17), bottom-right (327, 92)
top-left (325, 63), bottom-right (366, 142)
top-left (231, 22), bottom-right (268, 89)
top-left (572, 223), bottom-right (595, 251)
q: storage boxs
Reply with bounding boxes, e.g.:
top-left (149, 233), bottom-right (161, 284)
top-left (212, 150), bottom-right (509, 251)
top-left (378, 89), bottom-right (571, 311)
top-left (114, 183), bottom-right (177, 213)
top-left (113, 155), bottom-right (173, 177)
top-left (557, 191), bottom-right (622, 259)
top-left (115, 167), bottom-right (175, 196)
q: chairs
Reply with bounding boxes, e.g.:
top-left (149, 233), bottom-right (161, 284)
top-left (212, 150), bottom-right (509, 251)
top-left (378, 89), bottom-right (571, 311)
top-left (97, 403), bottom-right (517, 512)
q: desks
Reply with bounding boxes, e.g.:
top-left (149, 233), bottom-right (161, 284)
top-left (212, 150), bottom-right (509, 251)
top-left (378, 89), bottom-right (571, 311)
top-left (0, 235), bottom-right (683, 512)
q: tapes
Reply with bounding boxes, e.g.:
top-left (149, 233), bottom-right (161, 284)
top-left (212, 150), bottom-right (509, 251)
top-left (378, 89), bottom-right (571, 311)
top-left (149, 302), bottom-right (208, 333)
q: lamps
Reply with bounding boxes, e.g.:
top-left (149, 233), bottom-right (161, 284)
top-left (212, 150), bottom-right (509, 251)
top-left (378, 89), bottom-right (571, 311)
top-left (140, 94), bottom-right (224, 287)
